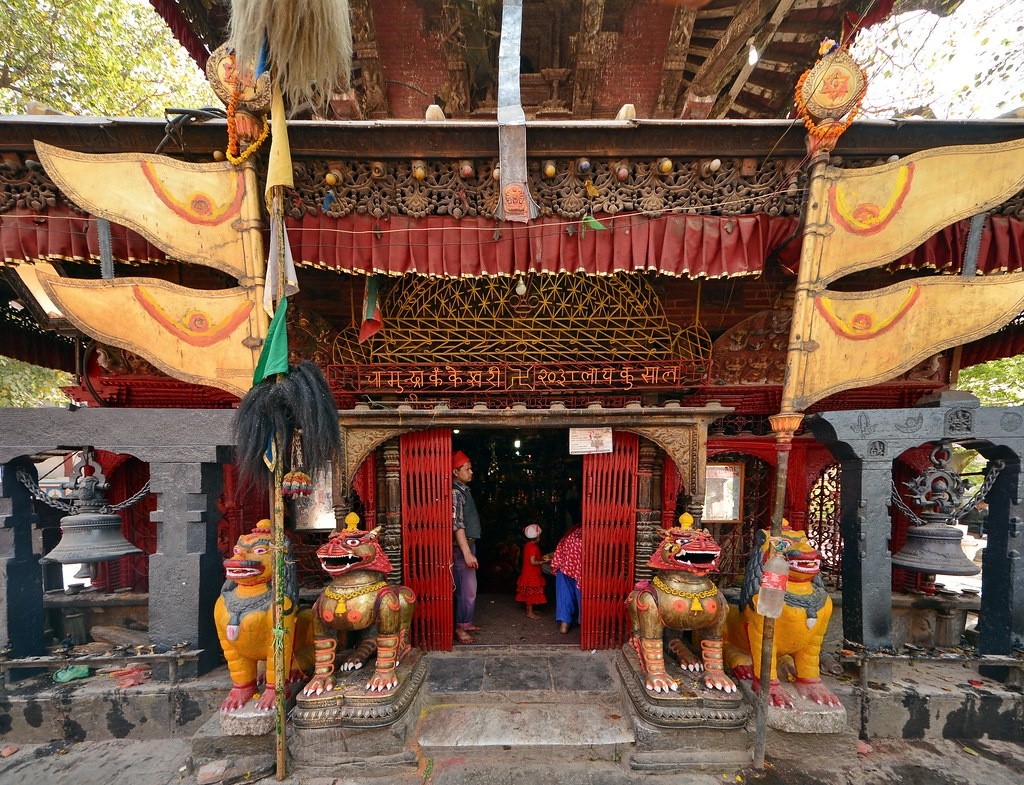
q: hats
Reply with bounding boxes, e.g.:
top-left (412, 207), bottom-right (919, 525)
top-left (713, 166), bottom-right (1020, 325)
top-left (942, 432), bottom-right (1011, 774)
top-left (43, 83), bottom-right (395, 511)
top-left (452, 451), bottom-right (469, 470)
top-left (524, 524), bottom-right (542, 538)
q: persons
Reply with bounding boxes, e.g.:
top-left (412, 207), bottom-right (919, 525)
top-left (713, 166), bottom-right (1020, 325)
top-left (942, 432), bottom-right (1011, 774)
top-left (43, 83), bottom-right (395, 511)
top-left (549, 525), bottom-right (583, 633)
top-left (452, 450), bottom-right (482, 644)
top-left (515, 524), bottom-right (551, 620)
